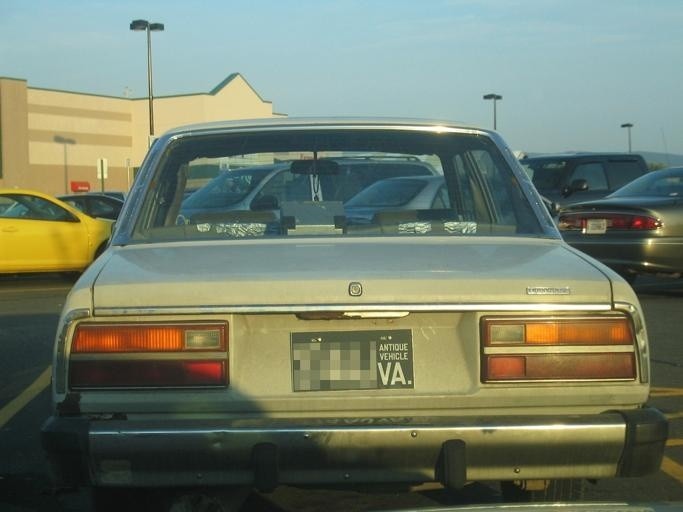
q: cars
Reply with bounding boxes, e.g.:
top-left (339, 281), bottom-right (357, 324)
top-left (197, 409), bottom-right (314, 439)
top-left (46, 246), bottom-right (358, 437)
top-left (343, 173), bottom-right (560, 238)
top-left (1, 147), bottom-right (441, 284)
top-left (37, 111), bottom-right (669, 507)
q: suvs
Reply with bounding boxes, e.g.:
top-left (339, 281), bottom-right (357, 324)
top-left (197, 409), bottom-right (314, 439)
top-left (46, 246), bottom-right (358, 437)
top-left (557, 166), bottom-right (682, 285)
top-left (518, 152), bottom-right (650, 214)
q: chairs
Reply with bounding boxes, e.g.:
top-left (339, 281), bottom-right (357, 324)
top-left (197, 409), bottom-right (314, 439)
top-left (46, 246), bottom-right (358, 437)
top-left (191, 210), bottom-right (468, 240)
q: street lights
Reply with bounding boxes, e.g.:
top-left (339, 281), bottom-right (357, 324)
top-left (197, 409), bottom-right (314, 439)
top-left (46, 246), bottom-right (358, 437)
top-left (620, 121), bottom-right (635, 154)
top-left (127, 17), bottom-right (164, 136)
top-left (481, 91), bottom-right (504, 133)
